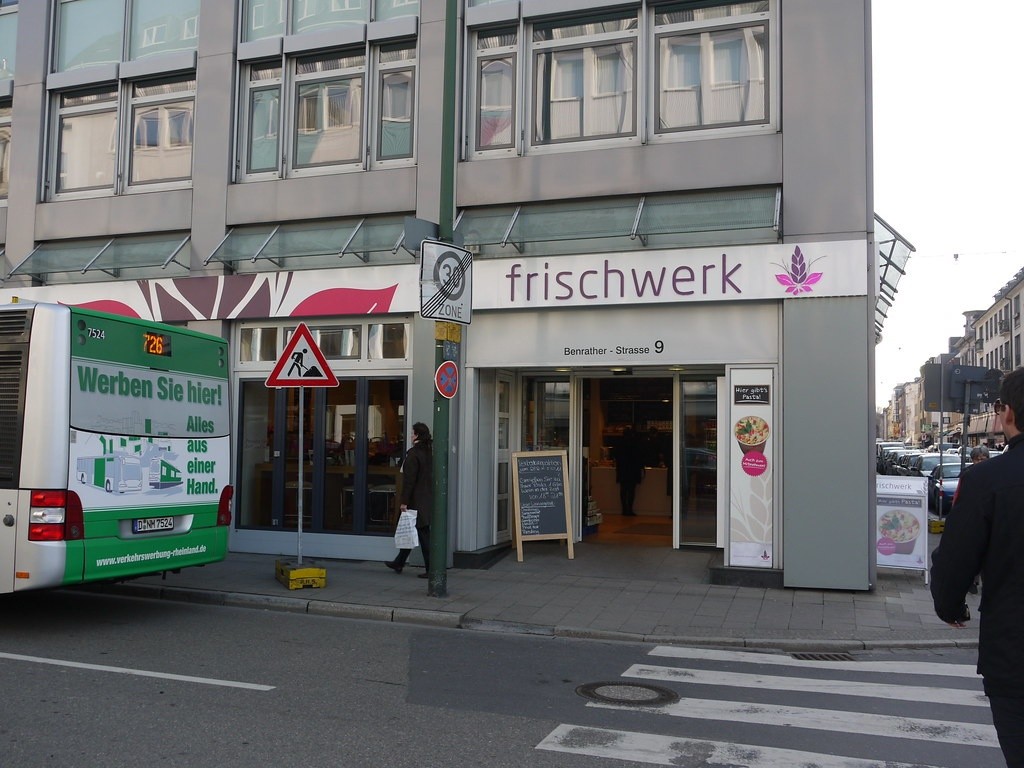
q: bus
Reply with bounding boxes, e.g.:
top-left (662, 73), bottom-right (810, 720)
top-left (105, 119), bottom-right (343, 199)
top-left (0, 293), bottom-right (234, 599)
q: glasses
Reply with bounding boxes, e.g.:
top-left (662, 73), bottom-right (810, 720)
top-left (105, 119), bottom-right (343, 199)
top-left (973, 458), bottom-right (985, 464)
top-left (994, 398), bottom-right (1006, 415)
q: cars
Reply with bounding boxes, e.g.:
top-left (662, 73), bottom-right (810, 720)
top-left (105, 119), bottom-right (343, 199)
top-left (928, 462), bottom-right (976, 513)
top-left (875, 440), bottom-right (1005, 479)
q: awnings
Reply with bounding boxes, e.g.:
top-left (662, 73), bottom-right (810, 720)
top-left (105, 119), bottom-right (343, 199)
top-left (943, 429), bottom-right (961, 437)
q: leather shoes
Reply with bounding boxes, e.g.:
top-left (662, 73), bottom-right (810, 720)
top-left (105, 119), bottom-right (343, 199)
top-left (417, 573), bottom-right (428, 578)
top-left (383, 561), bottom-right (404, 574)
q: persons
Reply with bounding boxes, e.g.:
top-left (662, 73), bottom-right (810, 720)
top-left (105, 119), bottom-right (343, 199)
top-left (384, 422), bottom-right (432, 578)
top-left (920, 433), bottom-right (933, 449)
top-left (610, 426), bottom-right (662, 516)
top-left (664, 432), bottom-right (691, 519)
top-left (330, 436), bottom-right (404, 523)
top-left (953, 440), bottom-right (960, 453)
top-left (930, 366), bottom-right (1024, 768)
top-left (952, 445), bottom-right (989, 594)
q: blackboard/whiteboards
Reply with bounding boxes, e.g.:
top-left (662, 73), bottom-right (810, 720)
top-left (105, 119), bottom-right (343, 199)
top-left (510, 450), bottom-right (574, 542)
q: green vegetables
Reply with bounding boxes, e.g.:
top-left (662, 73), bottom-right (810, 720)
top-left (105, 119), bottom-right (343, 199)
top-left (736, 418), bottom-right (753, 435)
top-left (880, 514), bottom-right (899, 530)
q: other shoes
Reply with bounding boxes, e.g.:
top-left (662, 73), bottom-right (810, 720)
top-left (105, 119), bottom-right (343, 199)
top-left (968, 583), bottom-right (978, 595)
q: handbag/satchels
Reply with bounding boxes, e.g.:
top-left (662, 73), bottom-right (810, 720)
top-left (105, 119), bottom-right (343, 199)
top-left (394, 509), bottom-right (419, 550)
